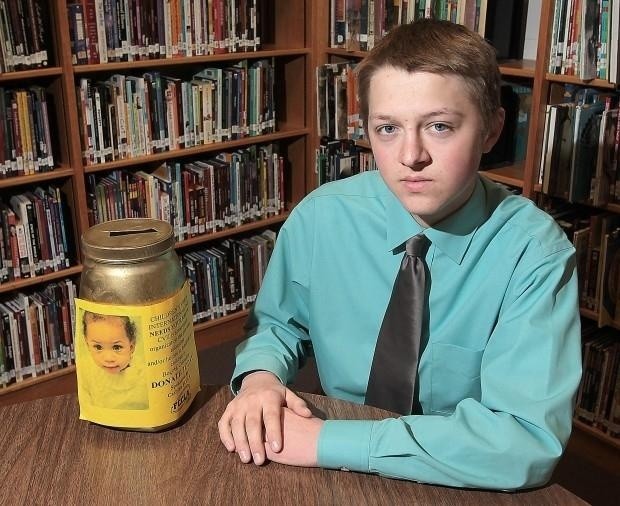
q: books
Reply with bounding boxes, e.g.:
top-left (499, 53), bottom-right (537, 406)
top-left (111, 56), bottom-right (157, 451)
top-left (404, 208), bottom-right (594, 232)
top-left (315, 0), bottom-right (620, 442)
top-left (1, 2), bottom-right (287, 390)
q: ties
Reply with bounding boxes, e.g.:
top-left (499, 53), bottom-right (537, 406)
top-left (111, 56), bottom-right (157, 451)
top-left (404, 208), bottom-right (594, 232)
top-left (364, 233), bottom-right (432, 416)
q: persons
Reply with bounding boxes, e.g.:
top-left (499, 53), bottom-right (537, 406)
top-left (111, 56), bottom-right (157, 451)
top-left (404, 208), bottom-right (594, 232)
top-left (75, 311), bottom-right (150, 410)
top-left (216, 16), bottom-right (586, 493)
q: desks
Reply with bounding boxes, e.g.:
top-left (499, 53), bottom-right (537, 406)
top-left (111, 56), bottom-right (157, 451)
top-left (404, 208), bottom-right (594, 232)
top-left (2, 386), bottom-right (591, 506)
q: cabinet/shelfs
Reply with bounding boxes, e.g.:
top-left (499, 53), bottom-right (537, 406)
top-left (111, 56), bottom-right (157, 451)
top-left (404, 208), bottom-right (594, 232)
top-left (1, 0), bottom-right (309, 408)
top-left (304, 0), bottom-right (620, 457)
